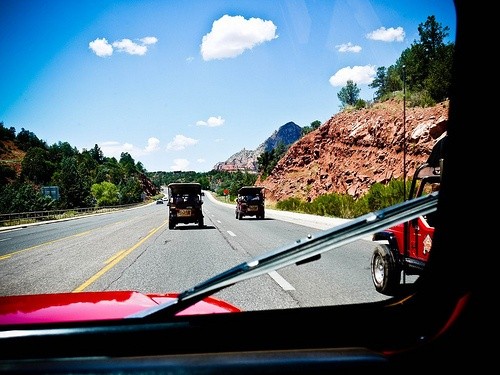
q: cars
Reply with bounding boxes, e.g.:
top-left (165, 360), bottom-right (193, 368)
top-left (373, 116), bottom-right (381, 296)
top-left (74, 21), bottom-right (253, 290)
top-left (156, 198), bottom-right (163, 204)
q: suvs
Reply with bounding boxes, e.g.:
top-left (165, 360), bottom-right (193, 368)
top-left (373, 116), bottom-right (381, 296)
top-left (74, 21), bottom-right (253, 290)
top-left (235, 186), bottom-right (265, 220)
top-left (371, 162), bottom-right (441, 293)
top-left (167, 183), bottom-right (206, 230)
